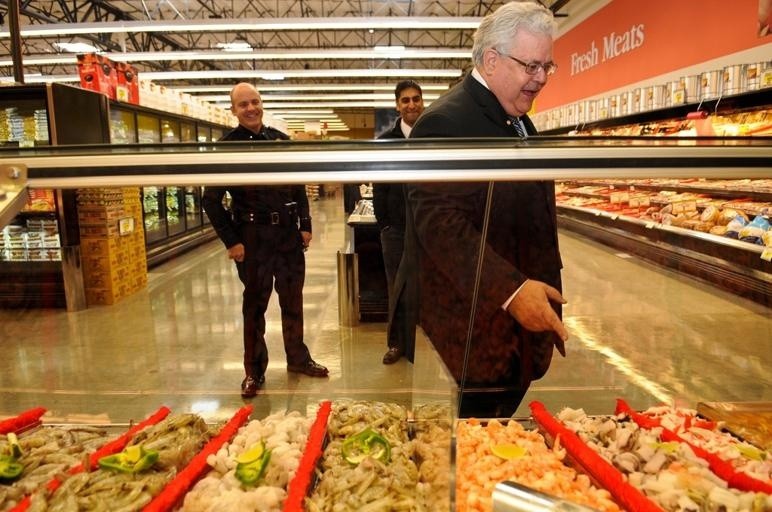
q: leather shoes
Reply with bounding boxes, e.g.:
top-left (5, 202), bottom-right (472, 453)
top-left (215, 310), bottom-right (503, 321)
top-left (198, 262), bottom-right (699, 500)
top-left (286, 358), bottom-right (329, 377)
top-left (241, 372), bottom-right (265, 398)
top-left (382, 343), bottom-right (405, 364)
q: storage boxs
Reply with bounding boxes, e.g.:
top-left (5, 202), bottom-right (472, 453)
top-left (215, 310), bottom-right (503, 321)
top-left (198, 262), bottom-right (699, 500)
top-left (78, 53), bottom-right (234, 126)
top-left (74, 202), bottom-right (151, 308)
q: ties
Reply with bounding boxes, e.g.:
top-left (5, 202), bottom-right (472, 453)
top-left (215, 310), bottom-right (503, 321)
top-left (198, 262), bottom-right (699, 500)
top-left (508, 114), bottom-right (526, 138)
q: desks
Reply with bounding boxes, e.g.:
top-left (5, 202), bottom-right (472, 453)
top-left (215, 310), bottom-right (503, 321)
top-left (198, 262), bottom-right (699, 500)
top-left (347, 198), bottom-right (393, 320)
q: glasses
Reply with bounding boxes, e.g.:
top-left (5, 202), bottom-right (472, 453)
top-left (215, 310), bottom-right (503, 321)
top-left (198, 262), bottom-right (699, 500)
top-left (490, 46), bottom-right (559, 76)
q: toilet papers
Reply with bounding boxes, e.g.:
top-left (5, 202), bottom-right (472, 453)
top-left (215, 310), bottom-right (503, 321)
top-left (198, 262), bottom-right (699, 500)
top-left (492, 480), bottom-right (600, 512)
top-left (686, 110), bottom-right (708, 119)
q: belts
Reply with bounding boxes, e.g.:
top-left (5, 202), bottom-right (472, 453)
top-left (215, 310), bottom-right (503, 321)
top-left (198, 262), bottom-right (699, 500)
top-left (234, 209), bottom-right (300, 226)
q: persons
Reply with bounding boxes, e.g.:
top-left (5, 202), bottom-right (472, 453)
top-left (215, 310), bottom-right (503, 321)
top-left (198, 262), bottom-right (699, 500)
top-left (371, 79), bottom-right (426, 366)
top-left (200, 81), bottom-right (329, 399)
top-left (400, 0), bottom-right (569, 429)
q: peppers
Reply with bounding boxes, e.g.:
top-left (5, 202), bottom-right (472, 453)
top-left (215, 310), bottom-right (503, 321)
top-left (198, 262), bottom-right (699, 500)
top-left (0, 456), bottom-right (23, 479)
top-left (96, 445), bottom-right (159, 473)
top-left (236, 441), bottom-right (270, 484)
top-left (342, 431), bottom-right (390, 467)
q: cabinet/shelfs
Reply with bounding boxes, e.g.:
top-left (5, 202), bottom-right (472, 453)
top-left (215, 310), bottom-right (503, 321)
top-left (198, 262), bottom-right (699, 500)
top-left (0, 79), bottom-right (110, 311)
top-left (106, 102), bottom-right (238, 265)
top-left (534, 84), bottom-right (767, 307)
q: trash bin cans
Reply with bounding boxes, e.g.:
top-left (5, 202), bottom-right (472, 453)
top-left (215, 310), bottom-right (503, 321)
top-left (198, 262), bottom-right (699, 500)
top-left (337, 250), bottom-right (360, 328)
top-left (61, 244), bottom-right (88, 313)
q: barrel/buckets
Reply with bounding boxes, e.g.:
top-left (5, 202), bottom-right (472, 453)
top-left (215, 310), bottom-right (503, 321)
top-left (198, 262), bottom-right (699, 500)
top-left (679, 61), bottom-right (772, 106)
top-left (529, 80), bottom-right (679, 132)
